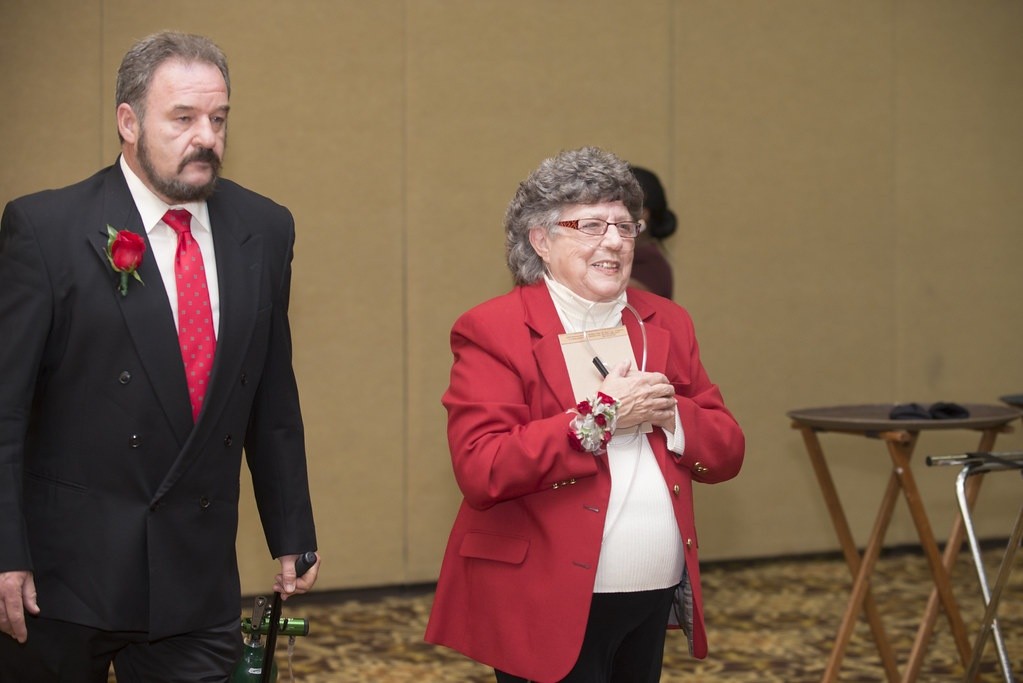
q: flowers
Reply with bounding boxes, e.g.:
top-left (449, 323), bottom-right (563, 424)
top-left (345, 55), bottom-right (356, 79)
top-left (573, 393), bottom-right (619, 452)
top-left (104, 227), bottom-right (148, 295)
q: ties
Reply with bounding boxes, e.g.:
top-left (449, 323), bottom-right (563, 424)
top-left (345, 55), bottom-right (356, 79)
top-left (160, 207), bottom-right (218, 425)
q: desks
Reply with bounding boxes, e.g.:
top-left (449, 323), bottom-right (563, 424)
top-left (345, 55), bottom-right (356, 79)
top-left (785, 402), bottom-right (1023, 683)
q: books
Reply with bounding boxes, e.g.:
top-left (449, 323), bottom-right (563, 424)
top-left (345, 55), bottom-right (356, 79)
top-left (557, 325), bottom-right (653, 434)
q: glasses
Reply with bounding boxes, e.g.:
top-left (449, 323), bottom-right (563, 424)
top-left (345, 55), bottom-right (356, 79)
top-left (888, 400), bottom-right (969, 418)
top-left (553, 217), bottom-right (641, 238)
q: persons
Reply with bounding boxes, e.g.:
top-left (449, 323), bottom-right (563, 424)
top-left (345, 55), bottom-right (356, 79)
top-left (1, 29), bottom-right (321, 682)
top-left (420, 148), bottom-right (745, 682)
top-left (631, 165), bottom-right (678, 302)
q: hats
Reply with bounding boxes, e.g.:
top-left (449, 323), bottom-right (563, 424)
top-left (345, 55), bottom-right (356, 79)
top-left (630, 166), bottom-right (678, 240)
